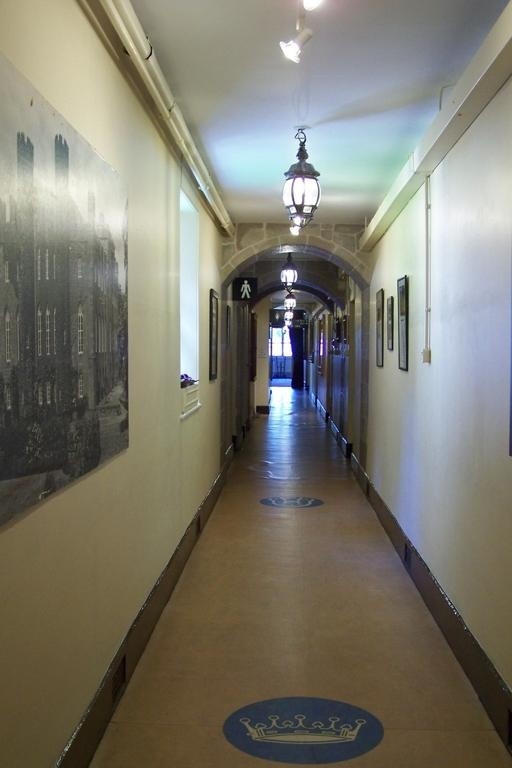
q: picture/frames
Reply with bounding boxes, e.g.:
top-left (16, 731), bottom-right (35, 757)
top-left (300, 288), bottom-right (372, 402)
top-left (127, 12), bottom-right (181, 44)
top-left (209, 288), bottom-right (219, 381)
top-left (374, 274), bottom-right (409, 373)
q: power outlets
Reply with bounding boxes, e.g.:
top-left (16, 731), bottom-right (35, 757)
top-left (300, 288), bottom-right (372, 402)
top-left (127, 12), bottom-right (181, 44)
top-left (282, 128), bottom-right (321, 228)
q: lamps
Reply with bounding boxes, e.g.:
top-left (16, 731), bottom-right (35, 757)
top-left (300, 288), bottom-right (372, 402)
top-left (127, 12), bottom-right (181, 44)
top-left (280, 252), bottom-right (298, 294)
